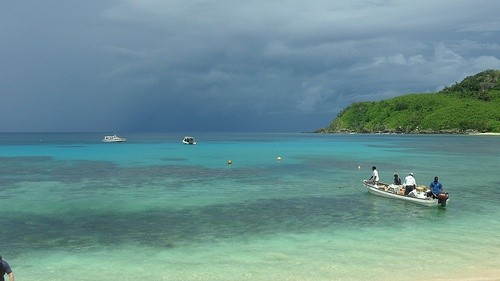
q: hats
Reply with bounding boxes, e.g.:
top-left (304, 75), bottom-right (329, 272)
top-left (409, 172), bottom-right (413, 176)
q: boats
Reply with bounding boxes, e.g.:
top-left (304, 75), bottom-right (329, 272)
top-left (103, 135), bottom-right (127, 143)
top-left (182, 136), bottom-right (196, 144)
top-left (362, 179), bottom-right (450, 208)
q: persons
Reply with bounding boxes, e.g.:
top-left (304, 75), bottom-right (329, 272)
top-left (0, 255), bottom-right (14, 281)
top-left (404, 172), bottom-right (416, 196)
top-left (369, 166), bottom-right (380, 186)
top-left (394, 174), bottom-right (401, 186)
top-left (426, 177), bottom-right (442, 200)
